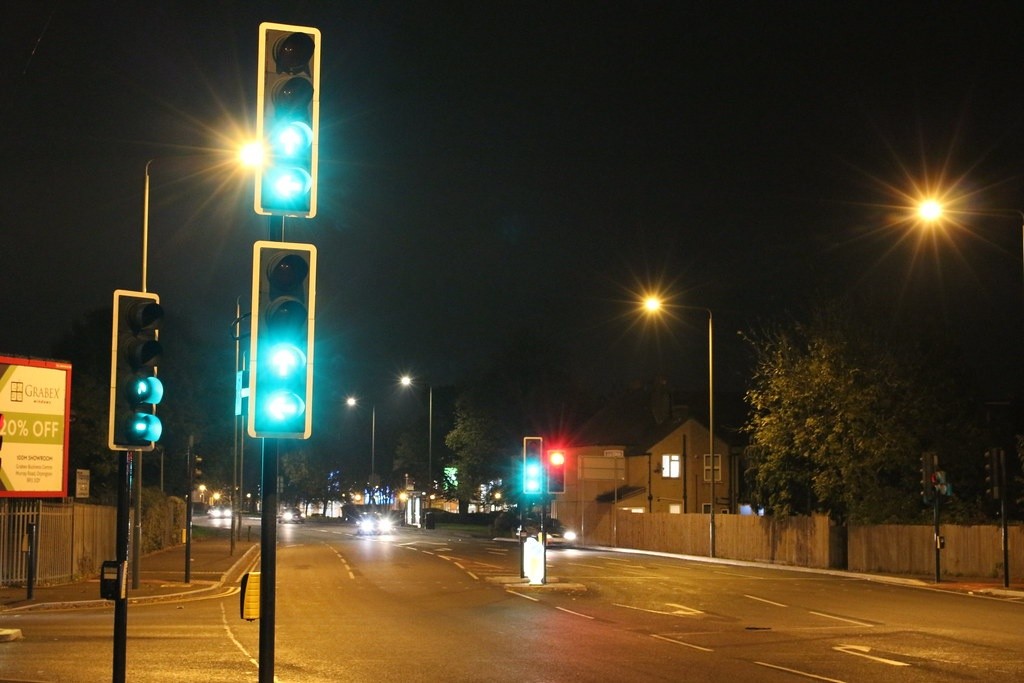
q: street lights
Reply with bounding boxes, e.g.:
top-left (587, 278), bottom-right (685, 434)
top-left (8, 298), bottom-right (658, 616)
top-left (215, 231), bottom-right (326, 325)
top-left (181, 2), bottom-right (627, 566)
top-left (400, 375), bottom-right (432, 510)
top-left (347, 398), bottom-right (377, 486)
top-left (641, 296), bottom-right (718, 558)
top-left (907, 191), bottom-right (1008, 588)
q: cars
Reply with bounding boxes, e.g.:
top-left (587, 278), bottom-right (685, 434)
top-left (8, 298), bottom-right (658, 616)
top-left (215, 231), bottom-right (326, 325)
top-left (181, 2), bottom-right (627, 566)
top-left (515, 517), bottom-right (574, 548)
top-left (209, 507), bottom-right (230, 518)
top-left (277, 507), bottom-right (306, 524)
top-left (359, 512), bottom-right (395, 533)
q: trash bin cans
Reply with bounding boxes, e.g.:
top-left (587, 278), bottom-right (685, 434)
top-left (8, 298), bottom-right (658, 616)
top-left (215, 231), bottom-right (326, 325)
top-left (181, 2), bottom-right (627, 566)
top-left (424, 512), bottom-right (435, 529)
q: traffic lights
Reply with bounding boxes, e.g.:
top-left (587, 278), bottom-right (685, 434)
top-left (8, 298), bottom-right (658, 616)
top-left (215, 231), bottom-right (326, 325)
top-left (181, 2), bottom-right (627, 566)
top-left (522, 436), bottom-right (541, 495)
top-left (253, 22), bottom-right (320, 221)
top-left (106, 289), bottom-right (167, 452)
top-left (984, 446), bottom-right (1001, 500)
top-left (930, 469), bottom-right (949, 500)
top-left (918, 451), bottom-right (936, 505)
top-left (547, 448), bottom-right (566, 495)
top-left (246, 238), bottom-right (316, 442)
